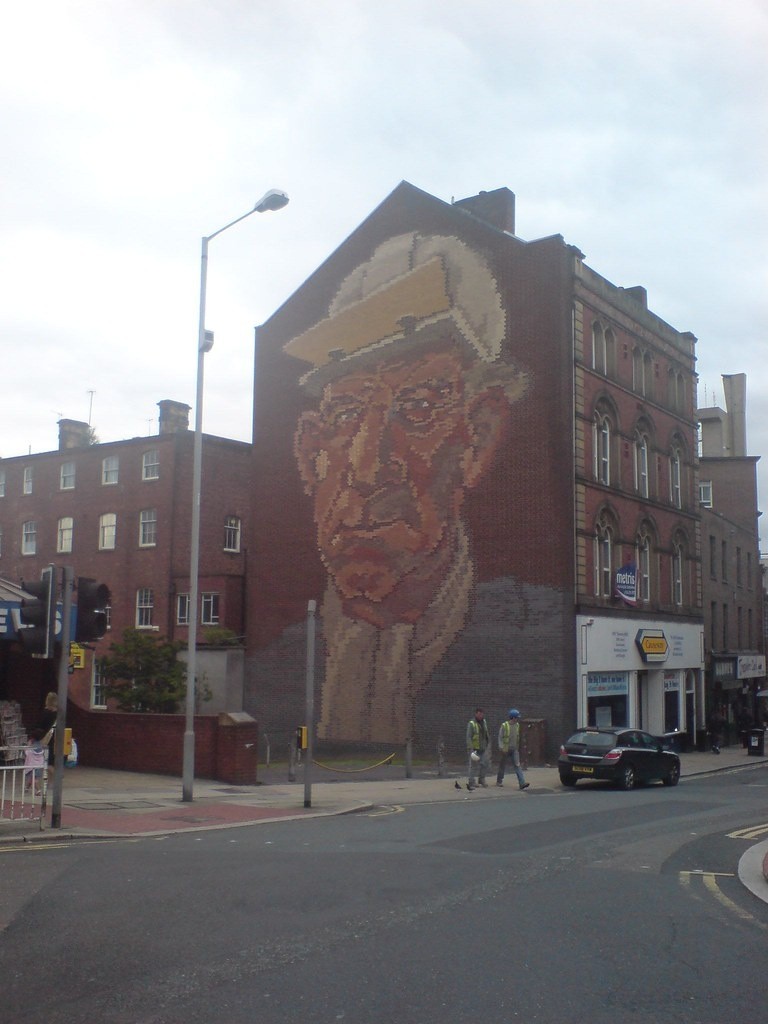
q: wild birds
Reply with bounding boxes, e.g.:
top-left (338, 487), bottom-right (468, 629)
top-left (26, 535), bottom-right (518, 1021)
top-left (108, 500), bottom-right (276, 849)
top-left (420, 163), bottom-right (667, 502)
top-left (454, 780), bottom-right (476, 793)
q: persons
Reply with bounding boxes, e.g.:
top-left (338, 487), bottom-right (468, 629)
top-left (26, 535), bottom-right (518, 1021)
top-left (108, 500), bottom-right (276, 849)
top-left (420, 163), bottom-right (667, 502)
top-left (23, 727), bottom-right (43, 796)
top-left (738, 707), bottom-right (754, 748)
top-left (707, 708), bottom-right (728, 754)
top-left (466, 708), bottom-right (491, 788)
top-left (42, 692), bottom-right (67, 779)
top-left (496, 709), bottom-right (529, 789)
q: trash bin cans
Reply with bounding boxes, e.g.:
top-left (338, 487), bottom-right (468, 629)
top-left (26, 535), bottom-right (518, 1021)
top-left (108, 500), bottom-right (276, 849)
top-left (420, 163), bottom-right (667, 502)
top-left (748, 729), bottom-right (764, 756)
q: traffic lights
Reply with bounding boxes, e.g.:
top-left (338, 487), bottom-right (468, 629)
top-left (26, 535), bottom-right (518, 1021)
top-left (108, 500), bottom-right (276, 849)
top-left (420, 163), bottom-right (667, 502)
top-left (20, 568), bottom-right (51, 658)
top-left (76, 576), bottom-right (111, 641)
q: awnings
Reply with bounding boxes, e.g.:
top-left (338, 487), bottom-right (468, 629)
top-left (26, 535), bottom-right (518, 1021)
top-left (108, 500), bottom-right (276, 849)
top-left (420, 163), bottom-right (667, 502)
top-left (713, 654), bottom-right (766, 681)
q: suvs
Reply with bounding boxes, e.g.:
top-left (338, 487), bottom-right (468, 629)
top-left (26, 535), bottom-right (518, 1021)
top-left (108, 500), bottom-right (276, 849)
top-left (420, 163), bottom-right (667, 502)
top-left (558, 728), bottom-right (681, 789)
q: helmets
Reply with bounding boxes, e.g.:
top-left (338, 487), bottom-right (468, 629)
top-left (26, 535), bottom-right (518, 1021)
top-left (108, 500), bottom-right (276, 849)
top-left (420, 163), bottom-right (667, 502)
top-left (470, 751), bottom-right (480, 762)
top-left (509, 710), bottom-right (520, 719)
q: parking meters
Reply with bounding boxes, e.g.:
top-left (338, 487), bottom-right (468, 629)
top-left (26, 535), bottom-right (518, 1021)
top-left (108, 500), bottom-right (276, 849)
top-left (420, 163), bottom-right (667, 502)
top-left (297, 726), bottom-right (307, 749)
top-left (53, 727), bottom-right (72, 755)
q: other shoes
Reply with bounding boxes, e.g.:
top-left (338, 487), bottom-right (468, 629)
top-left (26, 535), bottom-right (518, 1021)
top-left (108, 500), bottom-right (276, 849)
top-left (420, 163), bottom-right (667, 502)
top-left (716, 747), bottom-right (720, 755)
top-left (519, 782), bottom-right (529, 790)
top-left (478, 781), bottom-right (488, 787)
top-left (469, 782), bottom-right (479, 788)
top-left (496, 782), bottom-right (503, 787)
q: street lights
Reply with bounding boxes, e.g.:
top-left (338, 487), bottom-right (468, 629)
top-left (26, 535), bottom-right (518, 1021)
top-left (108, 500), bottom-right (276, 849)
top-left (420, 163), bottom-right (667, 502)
top-left (181, 189), bottom-right (290, 799)
